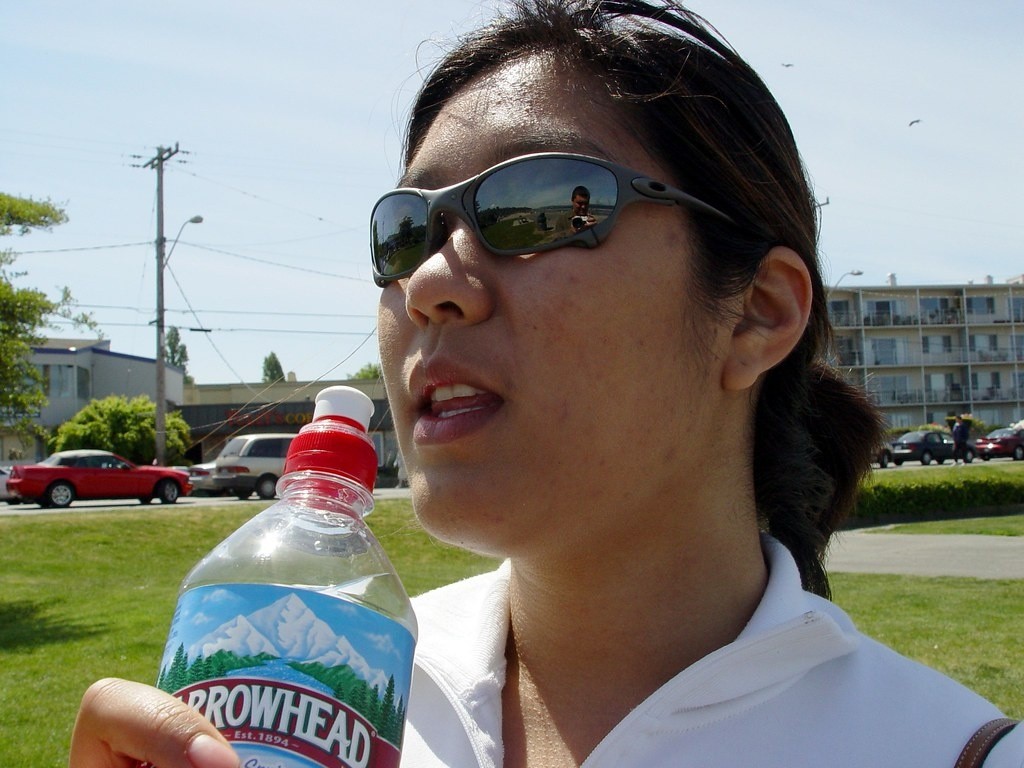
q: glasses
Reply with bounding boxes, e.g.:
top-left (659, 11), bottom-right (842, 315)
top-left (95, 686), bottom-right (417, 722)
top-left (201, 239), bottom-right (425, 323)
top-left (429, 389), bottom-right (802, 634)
top-left (369, 151), bottom-right (737, 287)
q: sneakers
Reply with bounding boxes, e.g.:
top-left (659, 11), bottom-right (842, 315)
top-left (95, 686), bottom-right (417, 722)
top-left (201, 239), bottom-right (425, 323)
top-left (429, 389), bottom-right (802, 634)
top-left (962, 462), bottom-right (965, 465)
top-left (952, 462), bottom-right (957, 466)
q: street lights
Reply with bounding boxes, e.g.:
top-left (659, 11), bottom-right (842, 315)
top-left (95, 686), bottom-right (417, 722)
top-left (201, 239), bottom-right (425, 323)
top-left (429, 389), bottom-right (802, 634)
top-left (154, 215), bottom-right (203, 467)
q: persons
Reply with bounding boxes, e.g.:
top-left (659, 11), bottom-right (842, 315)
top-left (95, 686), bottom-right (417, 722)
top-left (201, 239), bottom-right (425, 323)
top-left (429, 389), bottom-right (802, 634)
top-left (70, 0), bottom-right (1024, 768)
top-left (952, 416), bottom-right (969, 466)
top-left (554, 186), bottom-right (598, 239)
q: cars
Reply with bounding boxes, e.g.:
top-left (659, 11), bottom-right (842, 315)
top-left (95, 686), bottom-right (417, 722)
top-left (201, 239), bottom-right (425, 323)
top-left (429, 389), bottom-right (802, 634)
top-left (5, 450), bottom-right (193, 504)
top-left (189, 462), bottom-right (234, 496)
top-left (889, 430), bottom-right (976, 466)
top-left (870, 441), bottom-right (894, 468)
top-left (0, 467), bottom-right (22, 503)
top-left (975, 427), bottom-right (1024, 461)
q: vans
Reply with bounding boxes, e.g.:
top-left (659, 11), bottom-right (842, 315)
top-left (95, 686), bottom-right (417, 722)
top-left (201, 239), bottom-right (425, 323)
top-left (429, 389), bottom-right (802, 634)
top-left (213, 432), bottom-right (296, 501)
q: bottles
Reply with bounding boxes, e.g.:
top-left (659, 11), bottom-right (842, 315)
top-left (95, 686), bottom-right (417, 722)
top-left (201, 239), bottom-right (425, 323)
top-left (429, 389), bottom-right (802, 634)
top-left (154, 386), bottom-right (417, 768)
